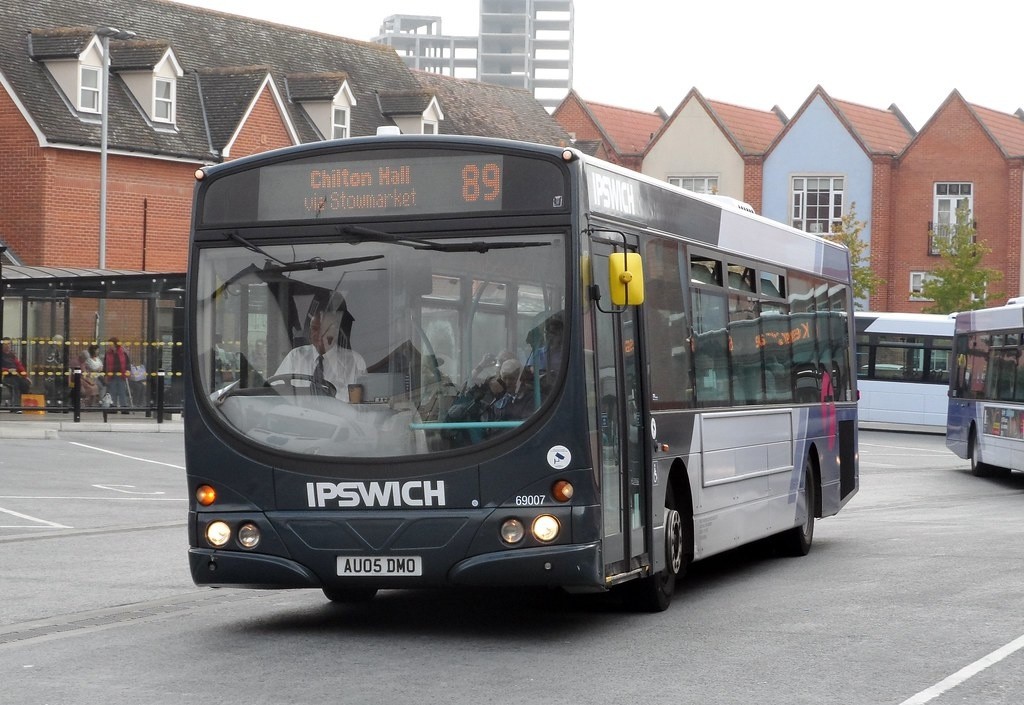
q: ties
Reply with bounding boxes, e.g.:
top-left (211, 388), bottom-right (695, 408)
top-left (309, 356), bottom-right (325, 388)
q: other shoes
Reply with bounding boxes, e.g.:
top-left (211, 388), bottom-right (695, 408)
top-left (16, 410), bottom-right (22, 414)
top-left (121, 411), bottom-right (130, 415)
top-left (107, 410), bottom-right (117, 415)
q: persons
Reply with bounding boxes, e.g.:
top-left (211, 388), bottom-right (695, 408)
top-left (460, 320), bottom-right (562, 434)
top-left (0, 337), bottom-right (31, 414)
top-left (44, 335), bottom-right (73, 413)
top-left (84, 346), bottom-right (106, 400)
top-left (130, 381), bottom-right (145, 407)
top-left (103, 338), bottom-right (131, 413)
top-left (69, 351), bottom-right (99, 406)
top-left (268, 312), bottom-right (363, 402)
top-left (696, 342), bottom-right (776, 403)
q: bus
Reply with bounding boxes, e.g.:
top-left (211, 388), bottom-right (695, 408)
top-left (829, 310), bottom-right (959, 427)
top-left (181, 134), bottom-right (864, 614)
top-left (946, 296), bottom-right (1024, 478)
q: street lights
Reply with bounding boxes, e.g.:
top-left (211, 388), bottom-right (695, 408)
top-left (92, 25), bottom-right (137, 364)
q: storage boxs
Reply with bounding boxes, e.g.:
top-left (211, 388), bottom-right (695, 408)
top-left (20, 394), bottom-right (46, 415)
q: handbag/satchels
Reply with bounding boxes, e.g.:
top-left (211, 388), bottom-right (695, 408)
top-left (132, 365), bottom-right (147, 382)
top-left (21, 395), bottom-right (46, 415)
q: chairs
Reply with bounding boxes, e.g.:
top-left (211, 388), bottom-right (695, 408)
top-left (929, 369), bottom-right (943, 380)
